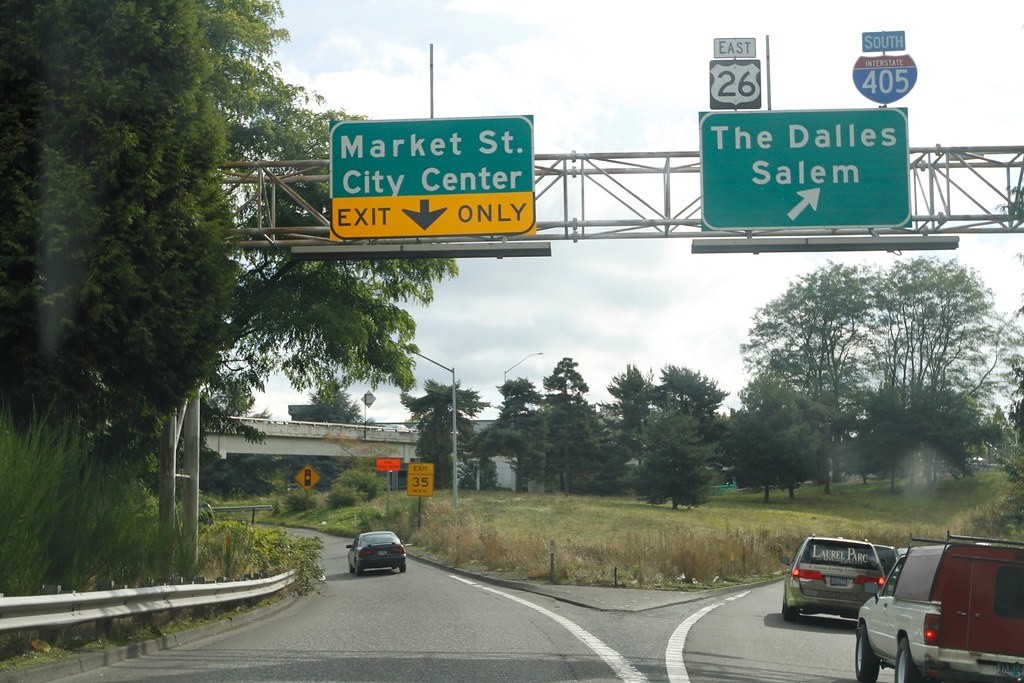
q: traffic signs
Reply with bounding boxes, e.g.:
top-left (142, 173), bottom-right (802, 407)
top-left (698, 107), bottom-right (912, 231)
top-left (328, 114), bottom-right (537, 242)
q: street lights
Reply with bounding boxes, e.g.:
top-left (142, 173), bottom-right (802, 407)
top-left (504, 353), bottom-right (543, 384)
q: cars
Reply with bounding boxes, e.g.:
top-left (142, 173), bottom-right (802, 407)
top-left (345, 531), bottom-right (406, 577)
top-left (779, 534), bottom-right (909, 623)
top-left (376, 424), bottom-right (409, 431)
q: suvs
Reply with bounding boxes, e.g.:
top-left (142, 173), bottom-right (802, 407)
top-left (855, 531), bottom-right (1024, 682)
